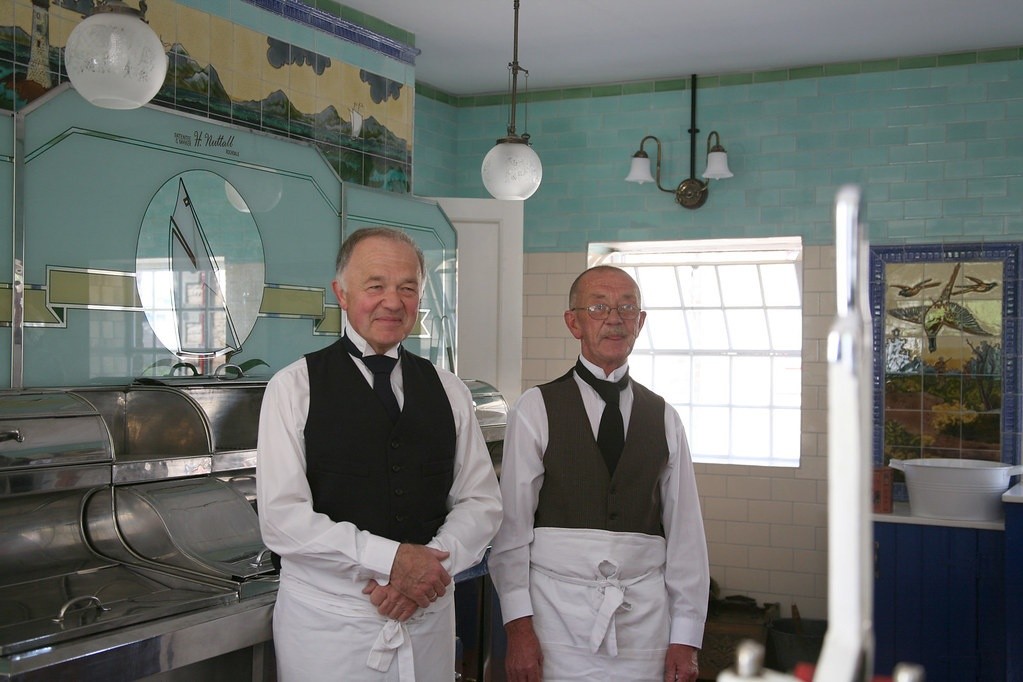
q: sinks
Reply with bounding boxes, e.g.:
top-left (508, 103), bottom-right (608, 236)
top-left (887, 457), bottom-right (1023, 521)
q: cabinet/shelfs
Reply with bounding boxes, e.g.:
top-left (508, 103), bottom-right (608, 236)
top-left (874, 520), bottom-right (1005, 682)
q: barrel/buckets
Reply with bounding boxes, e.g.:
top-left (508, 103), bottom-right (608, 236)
top-left (769, 618), bottom-right (828, 673)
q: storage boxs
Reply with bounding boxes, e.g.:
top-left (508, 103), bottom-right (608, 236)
top-left (697, 604), bottom-right (778, 680)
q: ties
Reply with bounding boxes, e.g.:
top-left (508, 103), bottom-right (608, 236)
top-left (575, 354), bottom-right (630, 477)
top-left (341, 326), bottom-right (403, 425)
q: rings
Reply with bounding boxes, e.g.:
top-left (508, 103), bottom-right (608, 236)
top-left (429, 593), bottom-right (437, 601)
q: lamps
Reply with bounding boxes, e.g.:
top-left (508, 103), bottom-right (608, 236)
top-left (64, 0), bottom-right (167, 109)
top-left (479, 0), bottom-right (542, 200)
top-left (625, 131), bottom-right (733, 209)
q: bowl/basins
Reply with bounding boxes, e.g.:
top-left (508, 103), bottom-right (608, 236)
top-left (889, 458), bottom-right (1023, 522)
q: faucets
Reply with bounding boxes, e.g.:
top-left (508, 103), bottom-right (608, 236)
top-left (0, 426), bottom-right (26, 443)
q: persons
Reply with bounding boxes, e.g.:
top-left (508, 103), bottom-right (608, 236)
top-left (485, 266), bottom-right (710, 682)
top-left (257, 227), bottom-right (503, 682)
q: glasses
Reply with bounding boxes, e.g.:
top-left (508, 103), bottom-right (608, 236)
top-left (571, 303), bottom-right (642, 320)
